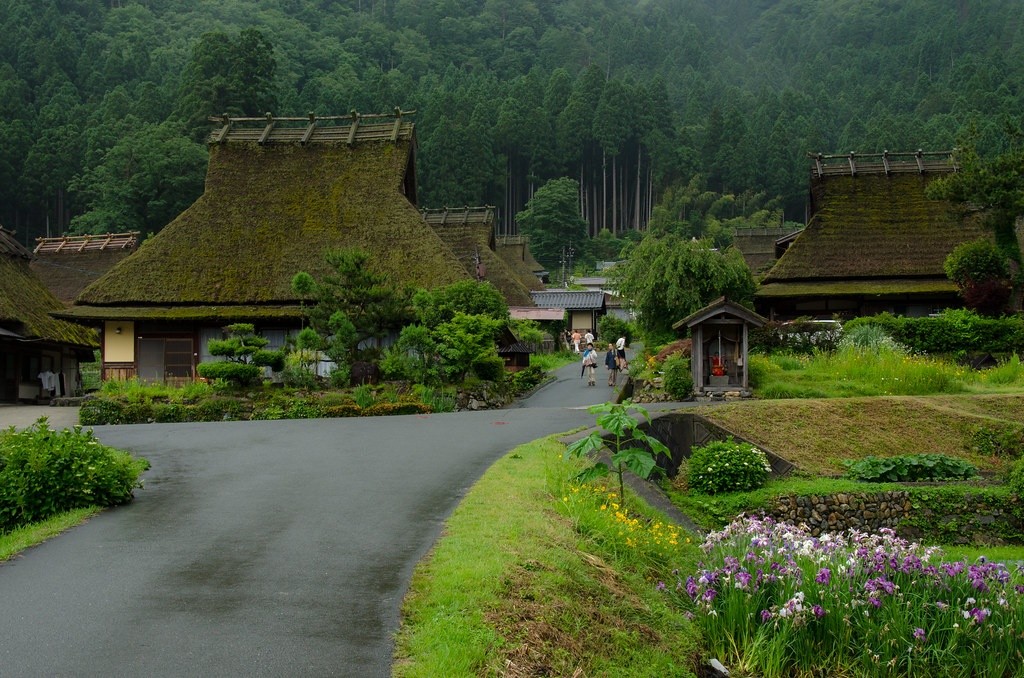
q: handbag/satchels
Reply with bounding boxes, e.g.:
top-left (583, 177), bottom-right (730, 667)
top-left (615, 349), bottom-right (619, 366)
top-left (592, 362), bottom-right (598, 368)
top-left (624, 361), bottom-right (628, 368)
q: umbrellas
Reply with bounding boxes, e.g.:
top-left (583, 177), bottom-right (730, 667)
top-left (580, 363), bottom-right (586, 379)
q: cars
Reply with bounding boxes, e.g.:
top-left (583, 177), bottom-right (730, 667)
top-left (770, 320), bottom-right (843, 351)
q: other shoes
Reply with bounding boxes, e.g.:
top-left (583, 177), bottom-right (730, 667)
top-left (588, 381), bottom-right (595, 386)
top-left (609, 383), bottom-right (616, 386)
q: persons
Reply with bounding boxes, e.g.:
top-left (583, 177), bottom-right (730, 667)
top-left (565, 329), bottom-right (571, 351)
top-left (616, 335), bottom-right (627, 369)
top-left (605, 343), bottom-right (621, 386)
top-left (571, 330), bottom-right (582, 354)
top-left (582, 343), bottom-right (598, 386)
top-left (585, 329), bottom-right (594, 344)
